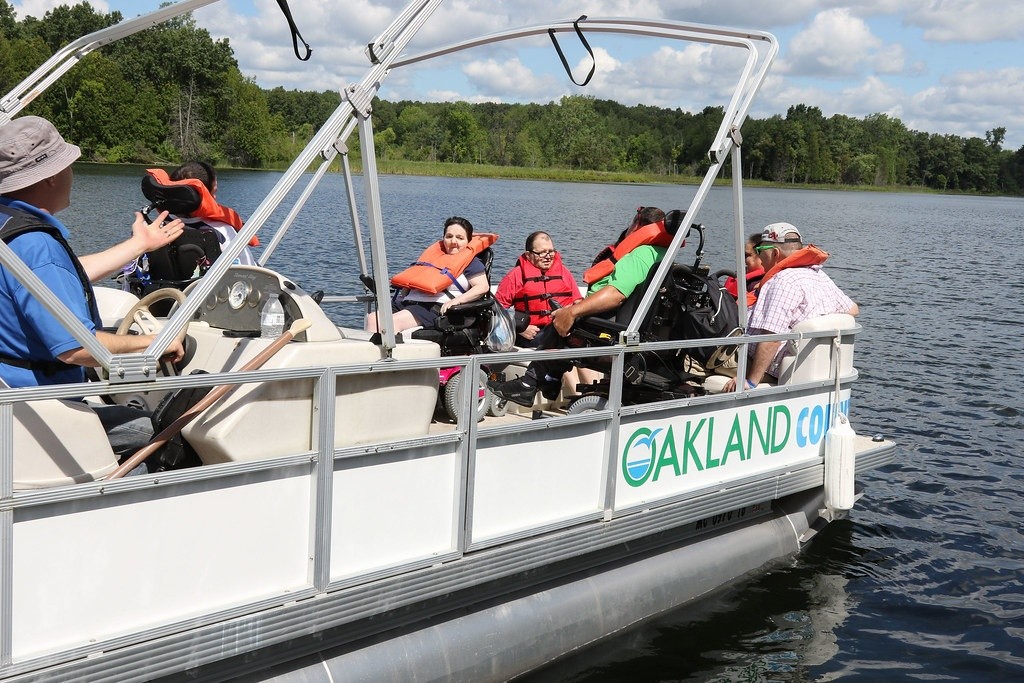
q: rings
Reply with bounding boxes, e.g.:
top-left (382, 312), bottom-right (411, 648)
top-left (167, 232), bottom-right (170, 236)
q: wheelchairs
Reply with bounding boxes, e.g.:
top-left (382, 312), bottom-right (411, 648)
top-left (111, 174), bottom-right (324, 317)
top-left (532, 223), bottom-right (735, 416)
top-left (359, 247), bottom-right (511, 421)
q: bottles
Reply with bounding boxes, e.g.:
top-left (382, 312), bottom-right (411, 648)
top-left (261, 294), bottom-right (284, 340)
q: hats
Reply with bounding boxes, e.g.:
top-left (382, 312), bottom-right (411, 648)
top-left (0, 116), bottom-right (82, 195)
top-left (753, 222), bottom-right (802, 249)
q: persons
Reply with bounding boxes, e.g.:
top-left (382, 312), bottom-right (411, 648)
top-left (723, 222), bottom-right (859, 392)
top-left (121, 161), bottom-right (257, 293)
top-left (495, 231), bottom-right (601, 396)
top-left (0, 115), bottom-right (185, 449)
top-left (365, 217), bottom-right (489, 335)
top-left (486, 206), bottom-right (673, 407)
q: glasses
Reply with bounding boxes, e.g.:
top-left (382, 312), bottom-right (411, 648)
top-left (745, 252), bottom-right (753, 259)
top-left (528, 249), bottom-right (557, 258)
top-left (755, 246), bottom-right (776, 255)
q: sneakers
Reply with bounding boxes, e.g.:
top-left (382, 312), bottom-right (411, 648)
top-left (485, 374), bottom-right (537, 408)
top-left (539, 374), bottom-right (563, 402)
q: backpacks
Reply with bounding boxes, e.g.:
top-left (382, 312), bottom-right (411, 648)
top-left (674, 274), bottom-right (743, 370)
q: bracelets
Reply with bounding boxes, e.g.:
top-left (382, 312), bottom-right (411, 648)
top-left (747, 379), bottom-right (755, 389)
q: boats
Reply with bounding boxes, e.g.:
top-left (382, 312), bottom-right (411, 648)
top-left (0, 0), bottom-right (899, 683)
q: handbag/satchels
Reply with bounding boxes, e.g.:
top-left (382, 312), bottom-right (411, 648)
top-left (484, 297), bottom-right (517, 353)
